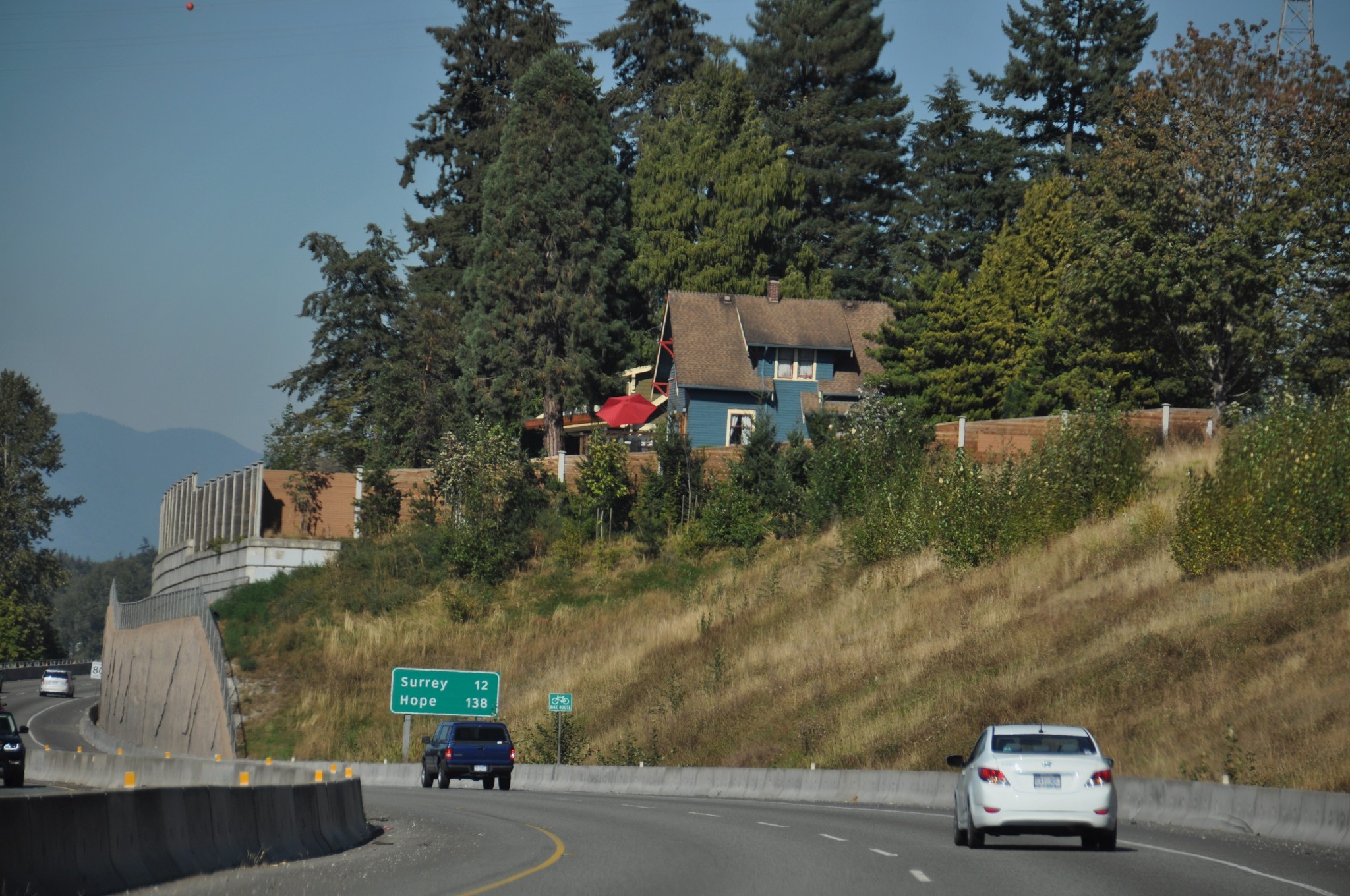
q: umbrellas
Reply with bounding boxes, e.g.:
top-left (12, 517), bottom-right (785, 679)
top-left (595, 394), bottom-right (658, 431)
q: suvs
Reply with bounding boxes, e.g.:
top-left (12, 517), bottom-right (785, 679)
top-left (420, 720), bottom-right (516, 791)
top-left (39, 669), bottom-right (77, 698)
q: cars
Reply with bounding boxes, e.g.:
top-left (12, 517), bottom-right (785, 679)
top-left (0, 703), bottom-right (30, 788)
top-left (945, 716), bottom-right (1118, 852)
